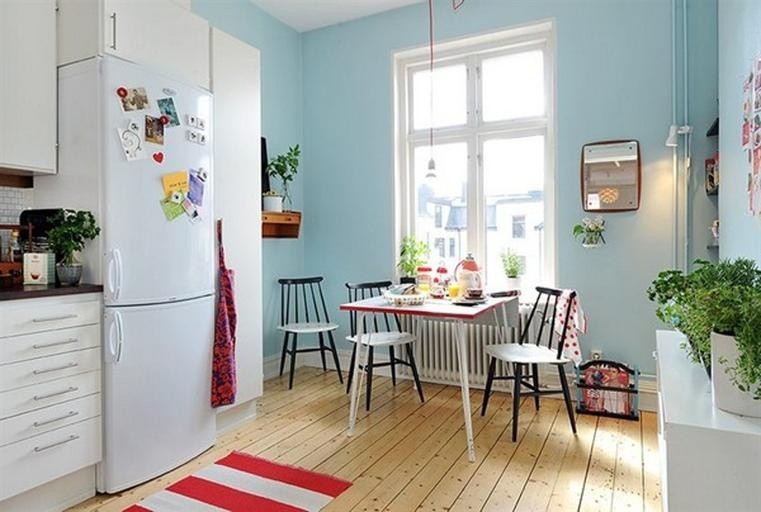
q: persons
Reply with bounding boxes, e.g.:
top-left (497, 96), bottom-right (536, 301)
top-left (594, 363), bottom-right (633, 416)
top-left (122, 90), bottom-right (149, 112)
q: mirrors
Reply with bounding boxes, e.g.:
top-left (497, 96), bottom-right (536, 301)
top-left (579, 140), bottom-right (640, 212)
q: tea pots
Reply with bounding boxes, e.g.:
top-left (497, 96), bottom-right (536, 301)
top-left (452, 255), bottom-right (481, 296)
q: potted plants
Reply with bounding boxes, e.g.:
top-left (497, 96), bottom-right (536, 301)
top-left (396, 233), bottom-right (430, 283)
top-left (669, 254), bottom-right (761, 419)
top-left (264, 142), bottom-right (301, 212)
top-left (498, 248), bottom-right (525, 291)
top-left (47, 208), bottom-right (100, 287)
top-left (571, 215), bottom-right (607, 247)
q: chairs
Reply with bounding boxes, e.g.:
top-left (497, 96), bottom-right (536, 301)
top-left (275, 275), bottom-right (343, 389)
top-left (481, 287), bottom-right (584, 442)
top-left (346, 278), bottom-right (425, 412)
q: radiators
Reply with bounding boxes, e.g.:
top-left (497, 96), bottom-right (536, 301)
top-left (393, 304), bottom-right (533, 396)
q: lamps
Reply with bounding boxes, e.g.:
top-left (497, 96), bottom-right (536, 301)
top-left (665, 124), bottom-right (695, 171)
top-left (597, 184), bottom-right (619, 204)
top-left (423, 1), bottom-right (443, 193)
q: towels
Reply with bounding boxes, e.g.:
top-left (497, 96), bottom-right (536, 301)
top-left (554, 289), bottom-right (588, 369)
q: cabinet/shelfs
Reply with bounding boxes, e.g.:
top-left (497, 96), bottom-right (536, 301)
top-left (212, 28), bottom-right (263, 435)
top-left (0, 292), bottom-right (102, 500)
top-left (0, 0), bottom-right (57, 177)
top-left (656, 329), bottom-right (760, 512)
top-left (704, 117), bottom-right (719, 247)
top-left (57, 0), bottom-right (212, 86)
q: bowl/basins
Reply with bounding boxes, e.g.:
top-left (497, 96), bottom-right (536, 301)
top-left (466, 287), bottom-right (482, 297)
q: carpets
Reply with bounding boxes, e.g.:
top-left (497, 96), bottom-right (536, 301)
top-left (118, 448), bottom-right (352, 512)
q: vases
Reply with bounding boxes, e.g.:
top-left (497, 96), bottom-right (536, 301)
top-left (263, 196), bottom-right (283, 213)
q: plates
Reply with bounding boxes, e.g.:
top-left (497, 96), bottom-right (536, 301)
top-left (459, 299), bottom-right (487, 304)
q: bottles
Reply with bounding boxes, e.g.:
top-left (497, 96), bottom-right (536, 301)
top-left (415, 266), bottom-right (447, 287)
top-left (264, 191), bottom-right (283, 211)
top-left (7, 237), bottom-right (23, 276)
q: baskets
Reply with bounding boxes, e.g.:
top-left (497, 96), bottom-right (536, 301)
top-left (382, 289), bottom-right (426, 306)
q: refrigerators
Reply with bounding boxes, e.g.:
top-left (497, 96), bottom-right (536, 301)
top-left (33, 52), bottom-right (216, 497)
